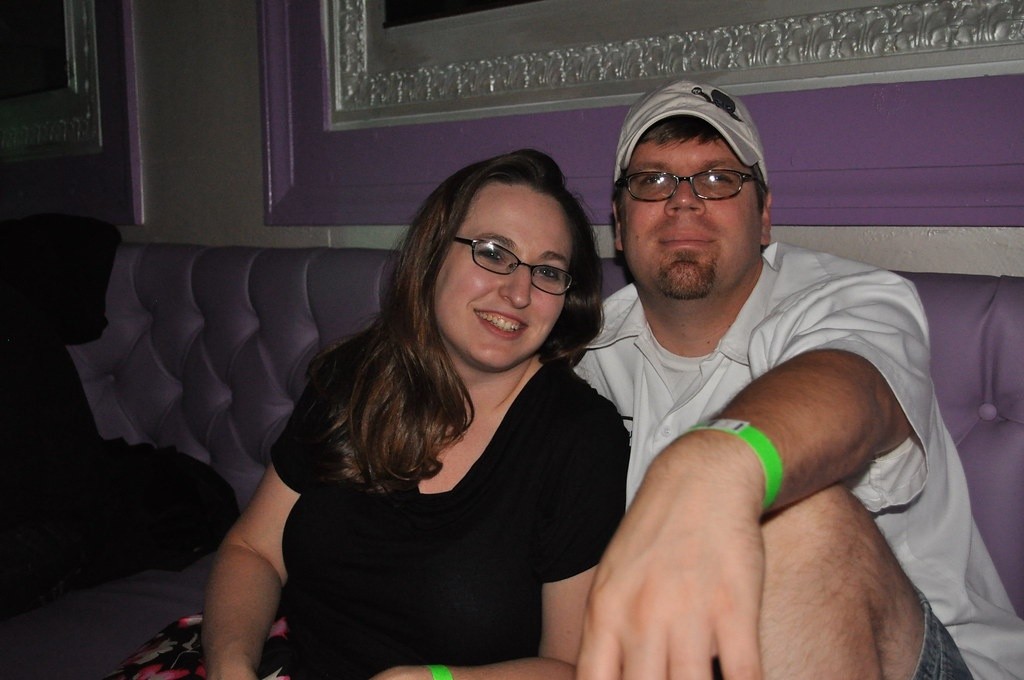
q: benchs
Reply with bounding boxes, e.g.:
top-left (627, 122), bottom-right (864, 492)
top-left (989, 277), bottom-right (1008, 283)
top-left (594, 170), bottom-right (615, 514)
top-left (0, 243), bottom-right (1024, 680)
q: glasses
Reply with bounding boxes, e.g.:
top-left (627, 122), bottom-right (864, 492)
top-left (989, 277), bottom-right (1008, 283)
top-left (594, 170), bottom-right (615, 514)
top-left (452, 235), bottom-right (574, 297)
top-left (613, 168), bottom-right (767, 203)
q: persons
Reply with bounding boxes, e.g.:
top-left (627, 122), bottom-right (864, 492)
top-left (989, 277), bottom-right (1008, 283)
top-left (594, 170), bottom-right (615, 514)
top-left (108, 149), bottom-right (632, 680)
top-left (571, 82), bottom-right (1024, 680)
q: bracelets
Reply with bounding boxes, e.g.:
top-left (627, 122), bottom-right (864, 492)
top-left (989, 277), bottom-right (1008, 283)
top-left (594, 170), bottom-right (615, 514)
top-left (426, 664), bottom-right (453, 680)
top-left (690, 419), bottom-right (782, 511)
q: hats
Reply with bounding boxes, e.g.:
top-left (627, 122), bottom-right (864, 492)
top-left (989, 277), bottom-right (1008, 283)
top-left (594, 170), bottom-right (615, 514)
top-left (614, 80), bottom-right (768, 188)
